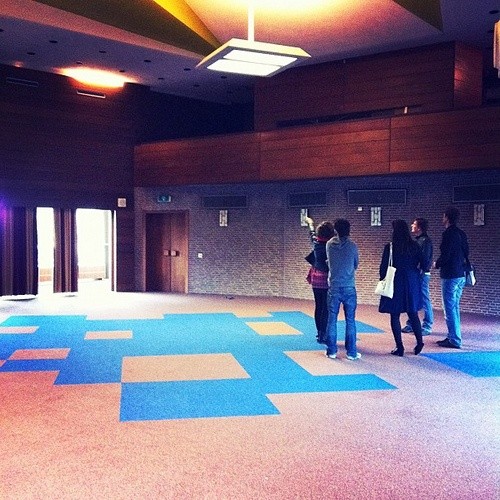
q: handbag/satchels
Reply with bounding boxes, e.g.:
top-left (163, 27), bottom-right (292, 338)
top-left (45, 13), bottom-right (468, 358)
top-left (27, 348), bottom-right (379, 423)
top-left (465, 270), bottom-right (476, 287)
top-left (374, 242), bottom-right (396, 299)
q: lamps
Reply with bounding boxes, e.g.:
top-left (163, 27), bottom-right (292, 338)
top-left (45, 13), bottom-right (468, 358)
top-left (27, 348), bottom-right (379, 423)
top-left (194, 0), bottom-right (312, 77)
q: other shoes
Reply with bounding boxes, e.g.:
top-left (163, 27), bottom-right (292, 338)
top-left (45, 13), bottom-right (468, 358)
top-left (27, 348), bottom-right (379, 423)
top-left (326, 352), bottom-right (336, 358)
top-left (391, 347), bottom-right (404, 357)
top-left (421, 328), bottom-right (432, 336)
top-left (402, 325), bottom-right (413, 333)
top-left (347, 353), bottom-right (361, 360)
top-left (414, 343), bottom-right (424, 356)
top-left (436, 338), bottom-right (460, 348)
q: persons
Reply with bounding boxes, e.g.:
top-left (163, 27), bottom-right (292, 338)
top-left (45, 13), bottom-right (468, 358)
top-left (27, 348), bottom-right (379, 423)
top-left (401, 217), bottom-right (435, 336)
top-left (304, 216), bottom-right (336, 344)
top-left (325, 219), bottom-right (362, 360)
top-left (430, 207), bottom-right (469, 348)
top-left (377, 218), bottom-right (431, 357)
